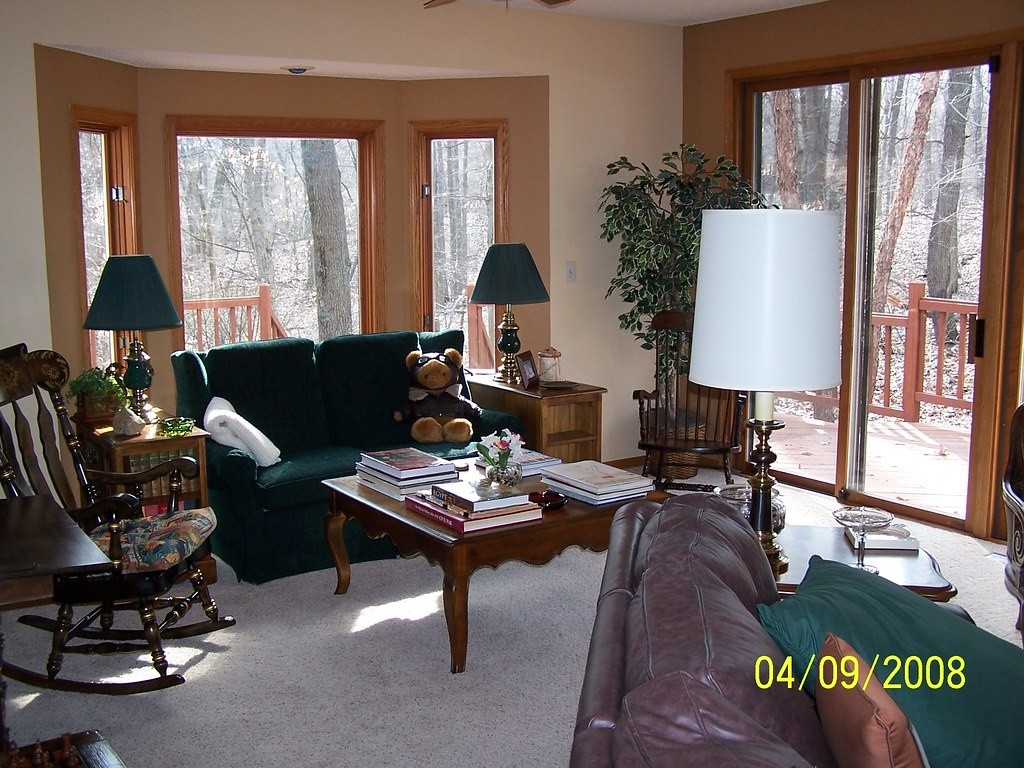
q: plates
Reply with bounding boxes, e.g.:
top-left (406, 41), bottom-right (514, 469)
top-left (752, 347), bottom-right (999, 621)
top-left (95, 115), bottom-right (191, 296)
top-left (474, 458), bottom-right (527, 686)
top-left (832, 506), bottom-right (895, 527)
top-left (540, 381), bottom-right (579, 389)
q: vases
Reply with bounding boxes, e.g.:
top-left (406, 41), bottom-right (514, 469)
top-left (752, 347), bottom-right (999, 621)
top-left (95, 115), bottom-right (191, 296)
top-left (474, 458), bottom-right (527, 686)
top-left (487, 461), bottom-right (522, 488)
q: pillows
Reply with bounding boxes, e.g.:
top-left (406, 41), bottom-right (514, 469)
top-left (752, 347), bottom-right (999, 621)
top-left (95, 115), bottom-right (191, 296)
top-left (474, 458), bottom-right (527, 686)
top-left (815, 631), bottom-right (922, 768)
top-left (757, 556), bottom-right (1024, 768)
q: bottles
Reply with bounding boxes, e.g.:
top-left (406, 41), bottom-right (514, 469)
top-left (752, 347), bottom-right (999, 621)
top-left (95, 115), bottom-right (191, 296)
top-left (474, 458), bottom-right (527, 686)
top-left (713, 484), bottom-right (785, 535)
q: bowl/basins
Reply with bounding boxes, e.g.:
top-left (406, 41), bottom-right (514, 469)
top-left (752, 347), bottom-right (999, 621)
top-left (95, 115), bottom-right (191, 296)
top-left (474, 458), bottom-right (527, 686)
top-left (158, 416), bottom-right (196, 437)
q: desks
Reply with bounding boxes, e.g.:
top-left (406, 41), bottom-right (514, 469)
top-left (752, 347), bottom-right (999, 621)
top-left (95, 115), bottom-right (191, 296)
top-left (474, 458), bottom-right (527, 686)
top-left (321, 458), bottom-right (677, 675)
top-left (770, 526), bottom-right (957, 605)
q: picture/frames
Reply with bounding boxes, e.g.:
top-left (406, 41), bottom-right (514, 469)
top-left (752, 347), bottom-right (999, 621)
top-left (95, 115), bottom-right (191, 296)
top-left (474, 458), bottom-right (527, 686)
top-left (515, 350), bottom-right (540, 391)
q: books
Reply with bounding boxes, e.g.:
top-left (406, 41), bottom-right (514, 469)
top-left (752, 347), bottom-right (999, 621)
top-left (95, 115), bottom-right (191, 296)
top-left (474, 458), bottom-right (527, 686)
top-left (475, 448), bottom-right (562, 477)
top-left (356, 447), bottom-right (463, 502)
top-left (130, 448), bottom-right (198, 516)
top-left (541, 460), bottom-right (655, 505)
top-left (405, 478), bottom-right (543, 533)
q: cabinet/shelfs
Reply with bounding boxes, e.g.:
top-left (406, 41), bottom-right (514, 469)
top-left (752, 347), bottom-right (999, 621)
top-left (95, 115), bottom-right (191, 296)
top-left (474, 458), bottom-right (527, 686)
top-left (465, 375), bottom-right (607, 465)
top-left (71, 405), bottom-right (218, 585)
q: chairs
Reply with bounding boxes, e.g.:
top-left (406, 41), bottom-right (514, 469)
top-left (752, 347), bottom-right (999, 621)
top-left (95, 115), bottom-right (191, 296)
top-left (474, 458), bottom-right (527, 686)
top-left (632, 310), bottom-right (747, 491)
top-left (0, 342), bottom-right (236, 697)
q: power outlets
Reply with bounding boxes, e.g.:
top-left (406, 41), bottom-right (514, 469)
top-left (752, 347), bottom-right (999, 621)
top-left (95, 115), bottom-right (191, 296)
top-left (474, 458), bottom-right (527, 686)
top-left (566, 260), bottom-right (576, 283)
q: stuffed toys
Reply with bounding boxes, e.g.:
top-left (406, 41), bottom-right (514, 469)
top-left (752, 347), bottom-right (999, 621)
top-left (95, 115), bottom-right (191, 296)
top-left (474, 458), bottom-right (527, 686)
top-left (394, 349), bottom-right (477, 443)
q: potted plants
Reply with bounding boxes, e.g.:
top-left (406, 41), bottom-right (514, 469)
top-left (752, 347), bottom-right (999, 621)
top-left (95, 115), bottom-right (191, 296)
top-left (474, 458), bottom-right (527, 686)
top-left (597, 143), bottom-right (779, 479)
top-left (70, 367), bottom-right (131, 425)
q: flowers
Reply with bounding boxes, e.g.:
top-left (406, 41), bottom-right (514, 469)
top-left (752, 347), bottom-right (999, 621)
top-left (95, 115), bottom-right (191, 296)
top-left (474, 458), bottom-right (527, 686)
top-left (476, 428), bottom-right (525, 467)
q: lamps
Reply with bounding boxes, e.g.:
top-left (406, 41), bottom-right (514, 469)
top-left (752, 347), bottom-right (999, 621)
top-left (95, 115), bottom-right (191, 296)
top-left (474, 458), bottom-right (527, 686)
top-left (82, 253), bottom-right (183, 423)
top-left (688, 208), bottom-right (841, 573)
top-left (468, 243), bottom-right (549, 383)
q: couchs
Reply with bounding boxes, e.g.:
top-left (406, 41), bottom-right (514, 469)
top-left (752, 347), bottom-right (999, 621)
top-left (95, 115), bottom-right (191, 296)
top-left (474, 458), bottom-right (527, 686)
top-left (570, 493), bottom-right (978, 768)
top-left (169, 330), bottom-right (522, 586)
